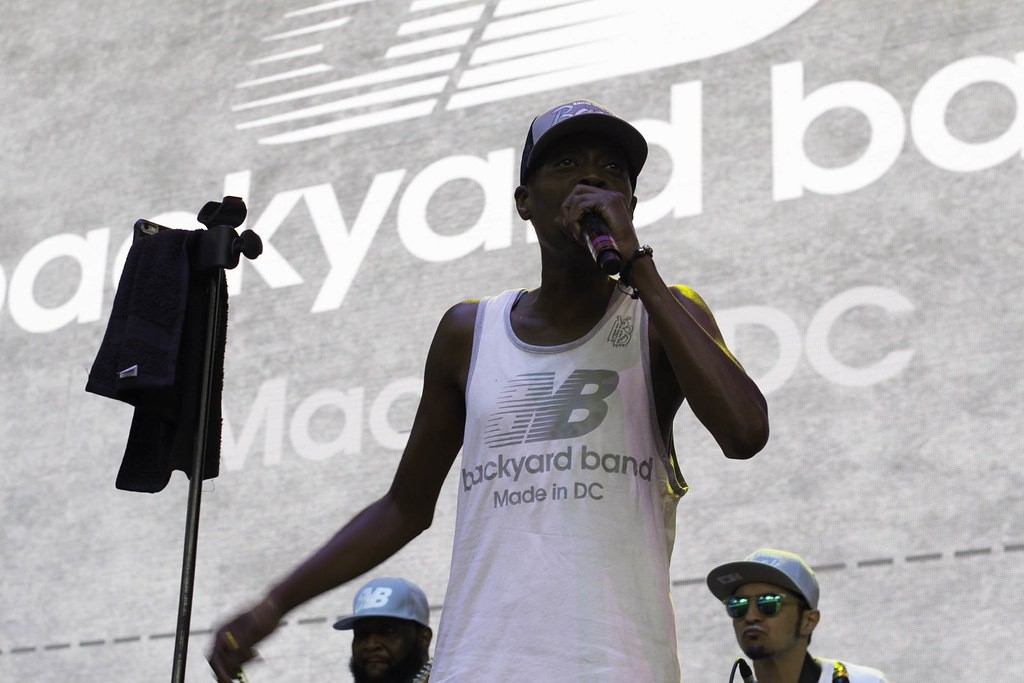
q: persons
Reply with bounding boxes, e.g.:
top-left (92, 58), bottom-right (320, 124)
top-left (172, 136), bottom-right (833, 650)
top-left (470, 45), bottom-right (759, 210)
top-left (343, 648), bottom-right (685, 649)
top-left (206, 98), bottom-right (769, 683)
top-left (708, 545), bottom-right (895, 683)
top-left (334, 574), bottom-right (436, 682)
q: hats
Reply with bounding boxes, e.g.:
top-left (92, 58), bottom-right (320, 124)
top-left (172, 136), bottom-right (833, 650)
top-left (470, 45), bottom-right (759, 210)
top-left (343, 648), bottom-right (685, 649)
top-left (333, 577), bottom-right (430, 630)
top-left (707, 548), bottom-right (820, 610)
top-left (520, 99), bottom-right (648, 195)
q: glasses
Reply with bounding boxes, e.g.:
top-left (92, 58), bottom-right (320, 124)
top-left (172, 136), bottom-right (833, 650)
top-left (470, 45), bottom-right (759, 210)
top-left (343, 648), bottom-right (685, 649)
top-left (723, 592), bottom-right (798, 619)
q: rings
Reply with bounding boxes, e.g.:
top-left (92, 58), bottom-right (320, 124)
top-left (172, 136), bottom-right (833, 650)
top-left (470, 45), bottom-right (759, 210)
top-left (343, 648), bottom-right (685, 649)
top-left (225, 631), bottom-right (239, 650)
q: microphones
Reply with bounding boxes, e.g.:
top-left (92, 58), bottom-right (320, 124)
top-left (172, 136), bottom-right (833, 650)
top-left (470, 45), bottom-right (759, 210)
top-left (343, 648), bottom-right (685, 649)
top-left (739, 659), bottom-right (755, 683)
top-left (580, 212), bottom-right (621, 275)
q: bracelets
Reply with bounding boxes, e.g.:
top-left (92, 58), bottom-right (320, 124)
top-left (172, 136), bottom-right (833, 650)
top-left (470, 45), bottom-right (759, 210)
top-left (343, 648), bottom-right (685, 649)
top-left (621, 243), bottom-right (655, 284)
top-left (264, 598), bottom-right (283, 618)
